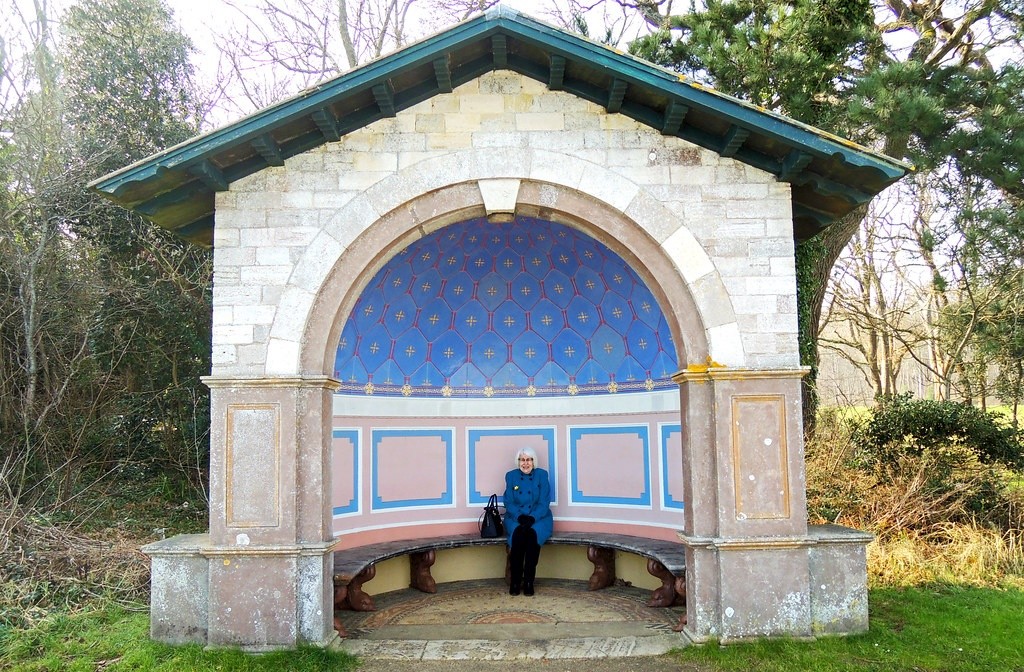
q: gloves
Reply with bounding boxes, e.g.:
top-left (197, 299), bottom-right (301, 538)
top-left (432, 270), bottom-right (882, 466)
top-left (517, 514), bottom-right (536, 527)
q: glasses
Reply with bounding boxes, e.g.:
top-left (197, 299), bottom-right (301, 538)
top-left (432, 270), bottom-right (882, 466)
top-left (518, 457), bottom-right (533, 462)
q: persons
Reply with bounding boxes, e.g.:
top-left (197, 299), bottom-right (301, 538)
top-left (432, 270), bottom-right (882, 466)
top-left (503, 447), bottom-right (554, 597)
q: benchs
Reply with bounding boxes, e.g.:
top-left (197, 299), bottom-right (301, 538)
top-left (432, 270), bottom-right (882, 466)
top-left (331, 531), bottom-right (688, 637)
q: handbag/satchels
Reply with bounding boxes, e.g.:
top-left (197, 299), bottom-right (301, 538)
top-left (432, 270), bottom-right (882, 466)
top-left (481, 494), bottom-right (503, 538)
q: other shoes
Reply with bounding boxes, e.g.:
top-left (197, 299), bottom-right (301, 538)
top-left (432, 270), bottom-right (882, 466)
top-left (523, 580), bottom-right (534, 596)
top-left (509, 580), bottom-right (522, 596)
